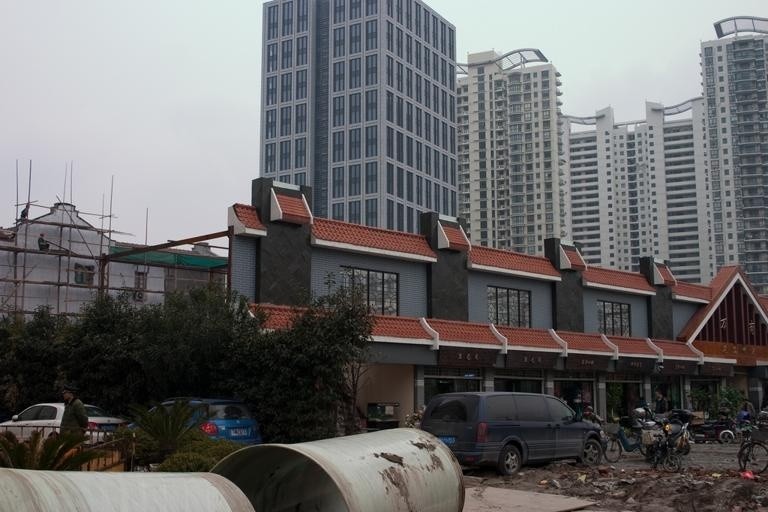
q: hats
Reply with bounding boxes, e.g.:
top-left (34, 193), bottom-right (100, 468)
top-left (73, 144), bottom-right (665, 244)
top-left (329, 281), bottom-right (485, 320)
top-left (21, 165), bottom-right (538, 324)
top-left (61, 385), bottom-right (79, 393)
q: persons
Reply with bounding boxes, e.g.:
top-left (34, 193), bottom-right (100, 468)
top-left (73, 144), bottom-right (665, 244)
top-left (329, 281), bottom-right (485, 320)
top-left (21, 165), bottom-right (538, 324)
top-left (653, 385), bottom-right (670, 425)
top-left (58, 384), bottom-right (90, 438)
top-left (742, 397), bottom-right (756, 422)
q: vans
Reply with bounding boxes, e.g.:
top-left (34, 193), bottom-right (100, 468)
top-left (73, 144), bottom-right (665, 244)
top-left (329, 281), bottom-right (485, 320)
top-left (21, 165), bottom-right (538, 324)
top-left (414, 389), bottom-right (603, 478)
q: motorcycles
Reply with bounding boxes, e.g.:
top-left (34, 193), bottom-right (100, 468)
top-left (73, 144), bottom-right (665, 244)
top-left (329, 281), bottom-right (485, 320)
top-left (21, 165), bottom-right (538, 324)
top-left (599, 404), bottom-right (747, 474)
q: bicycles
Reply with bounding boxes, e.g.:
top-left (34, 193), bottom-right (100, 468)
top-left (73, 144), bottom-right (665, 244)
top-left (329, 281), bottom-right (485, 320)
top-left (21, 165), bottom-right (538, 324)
top-left (734, 410), bottom-right (767, 475)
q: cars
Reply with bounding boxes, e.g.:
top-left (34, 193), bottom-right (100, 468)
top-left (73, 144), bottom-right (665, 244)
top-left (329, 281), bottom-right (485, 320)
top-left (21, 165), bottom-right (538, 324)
top-left (0, 399), bottom-right (136, 449)
top-left (119, 396), bottom-right (265, 466)
